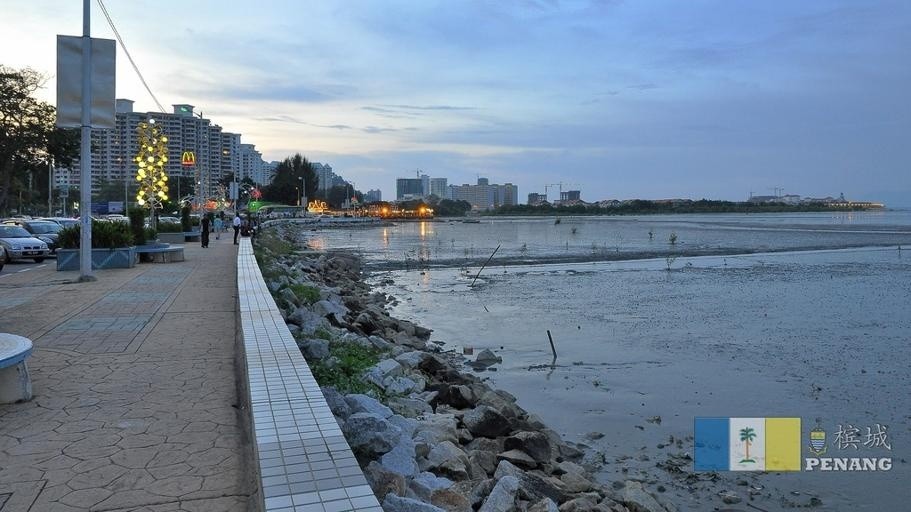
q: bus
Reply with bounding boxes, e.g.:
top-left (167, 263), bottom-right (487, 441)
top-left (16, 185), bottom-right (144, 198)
top-left (259, 205), bottom-right (302, 221)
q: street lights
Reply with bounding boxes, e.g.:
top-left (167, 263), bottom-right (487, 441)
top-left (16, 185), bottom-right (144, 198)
top-left (296, 186), bottom-right (300, 206)
top-left (298, 176), bottom-right (307, 214)
top-left (251, 187), bottom-right (258, 217)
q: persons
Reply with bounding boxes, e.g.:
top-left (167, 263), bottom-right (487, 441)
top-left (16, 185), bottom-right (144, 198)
top-left (199, 212), bottom-right (241, 247)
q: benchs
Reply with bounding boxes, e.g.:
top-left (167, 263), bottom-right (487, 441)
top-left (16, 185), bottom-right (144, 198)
top-left (135, 245), bottom-right (185, 265)
top-left (183, 231), bottom-right (204, 243)
top-left (0, 329), bottom-right (37, 407)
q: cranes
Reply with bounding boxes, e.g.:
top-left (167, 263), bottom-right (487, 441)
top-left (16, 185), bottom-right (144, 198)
top-left (406, 168), bottom-right (424, 178)
top-left (549, 181), bottom-right (584, 192)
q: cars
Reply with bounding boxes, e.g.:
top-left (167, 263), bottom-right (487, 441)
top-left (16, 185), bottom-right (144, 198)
top-left (0, 224), bottom-right (50, 263)
top-left (5, 220), bottom-right (67, 253)
top-left (91, 212), bottom-right (203, 232)
top-left (40, 217), bottom-right (81, 233)
top-left (0, 214), bottom-right (37, 222)
